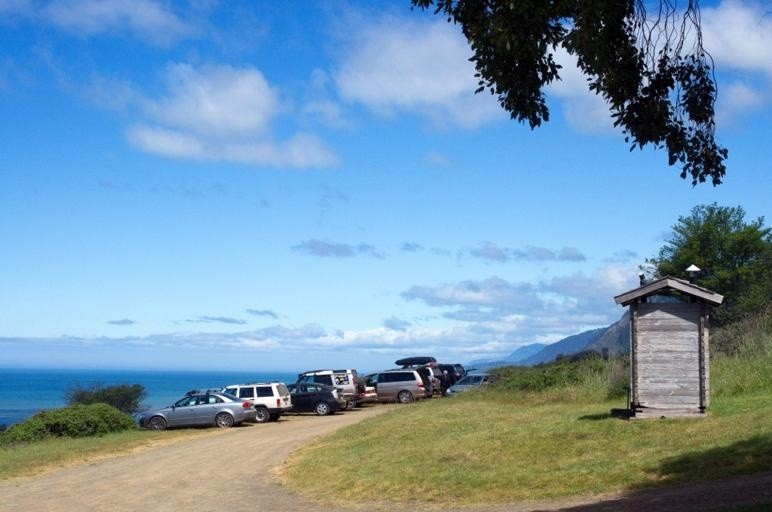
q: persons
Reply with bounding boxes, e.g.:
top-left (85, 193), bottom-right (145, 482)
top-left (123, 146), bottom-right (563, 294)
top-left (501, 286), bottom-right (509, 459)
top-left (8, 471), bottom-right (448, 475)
top-left (434, 370), bottom-right (448, 396)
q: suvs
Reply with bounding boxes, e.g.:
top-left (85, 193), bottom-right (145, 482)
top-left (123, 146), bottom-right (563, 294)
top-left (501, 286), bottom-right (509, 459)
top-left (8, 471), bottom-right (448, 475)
top-left (190, 380), bottom-right (294, 423)
top-left (295, 369), bottom-right (361, 411)
top-left (357, 356), bottom-right (466, 407)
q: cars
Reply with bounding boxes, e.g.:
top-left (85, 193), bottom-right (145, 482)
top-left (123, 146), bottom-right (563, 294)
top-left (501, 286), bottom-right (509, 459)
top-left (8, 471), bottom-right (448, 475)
top-left (137, 383), bottom-right (258, 431)
top-left (285, 382), bottom-right (347, 416)
top-left (444, 373), bottom-right (492, 398)
